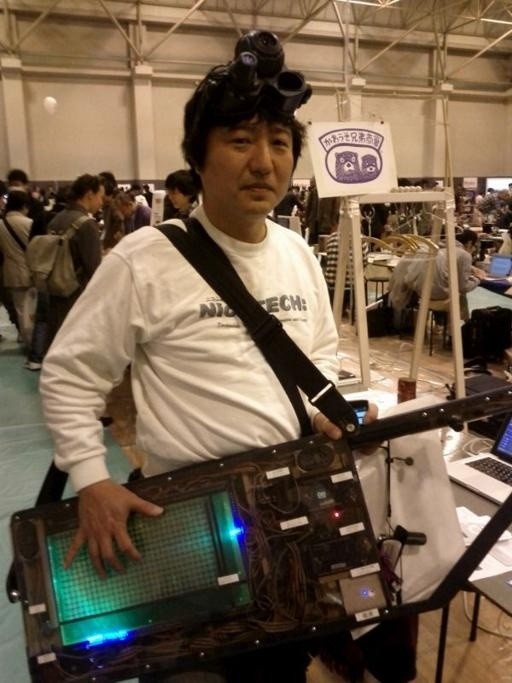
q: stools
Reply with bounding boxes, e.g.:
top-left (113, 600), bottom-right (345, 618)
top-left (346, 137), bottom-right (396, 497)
top-left (418, 298), bottom-right (450, 356)
top-left (366, 275), bottom-right (389, 306)
top-left (433, 568), bottom-right (511, 682)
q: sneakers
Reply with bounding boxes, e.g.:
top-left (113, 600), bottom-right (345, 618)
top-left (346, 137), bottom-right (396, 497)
top-left (23, 360), bottom-right (40, 370)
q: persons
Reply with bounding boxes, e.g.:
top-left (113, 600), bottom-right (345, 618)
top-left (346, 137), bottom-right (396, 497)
top-left (270, 174), bottom-right (512, 371)
top-left (37, 60), bottom-right (384, 683)
top-left (2, 169), bottom-right (202, 367)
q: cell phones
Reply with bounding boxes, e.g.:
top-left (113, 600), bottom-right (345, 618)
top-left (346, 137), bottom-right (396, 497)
top-left (346, 400), bottom-right (368, 426)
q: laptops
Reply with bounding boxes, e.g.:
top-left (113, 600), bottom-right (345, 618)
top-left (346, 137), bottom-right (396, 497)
top-left (446, 411), bottom-right (512, 507)
top-left (487, 254), bottom-right (512, 278)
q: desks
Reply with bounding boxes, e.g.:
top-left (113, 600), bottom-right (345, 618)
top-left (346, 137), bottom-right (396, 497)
top-left (364, 250), bottom-right (401, 272)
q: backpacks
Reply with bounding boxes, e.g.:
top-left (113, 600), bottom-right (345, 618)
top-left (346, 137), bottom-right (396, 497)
top-left (24, 215), bottom-right (93, 299)
